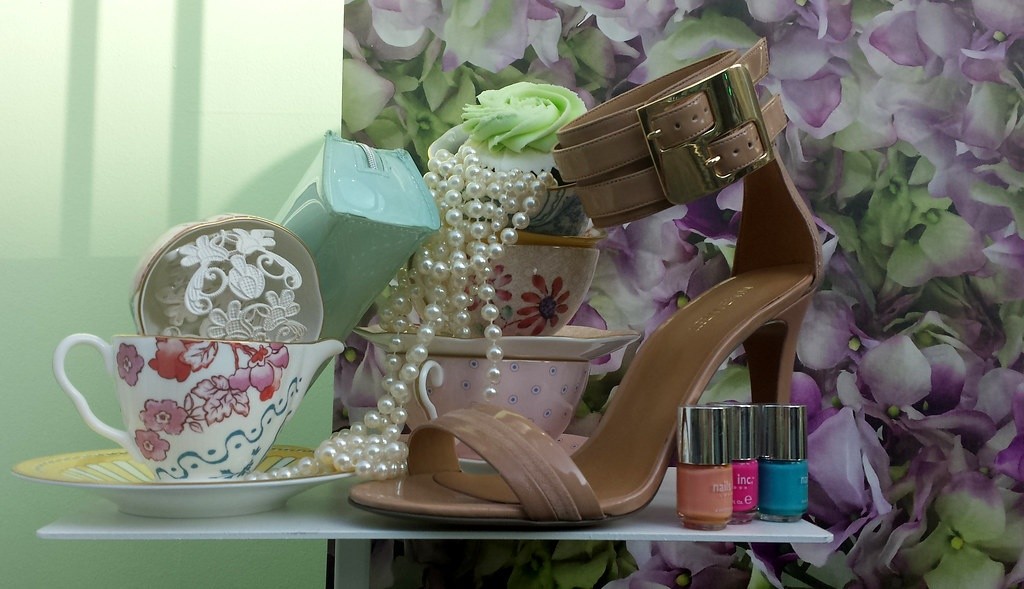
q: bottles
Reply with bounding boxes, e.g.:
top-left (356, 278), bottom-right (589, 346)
top-left (676, 405), bottom-right (734, 527)
top-left (705, 401), bottom-right (756, 524)
top-left (756, 403), bottom-right (808, 523)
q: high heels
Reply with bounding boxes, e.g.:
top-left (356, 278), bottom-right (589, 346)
top-left (349, 38), bottom-right (821, 529)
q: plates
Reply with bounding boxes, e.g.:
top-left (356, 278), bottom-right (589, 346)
top-left (351, 322), bottom-right (643, 364)
top-left (11, 446), bottom-right (355, 521)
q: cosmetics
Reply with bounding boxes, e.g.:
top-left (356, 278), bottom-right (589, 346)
top-left (759, 405), bottom-right (809, 523)
top-left (709, 404), bottom-right (761, 524)
top-left (678, 405), bottom-right (734, 531)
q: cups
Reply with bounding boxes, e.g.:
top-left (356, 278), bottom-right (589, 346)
top-left (385, 355), bottom-right (589, 460)
top-left (463, 134), bottom-right (593, 238)
top-left (51, 331), bottom-right (344, 483)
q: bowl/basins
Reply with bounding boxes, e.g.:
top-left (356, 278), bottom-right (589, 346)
top-left (128, 216), bottom-right (325, 343)
top-left (489, 248), bottom-right (600, 333)
